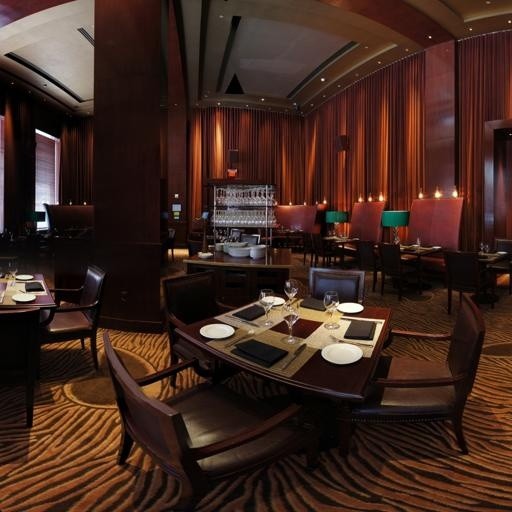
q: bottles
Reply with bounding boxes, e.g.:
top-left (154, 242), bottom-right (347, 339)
top-left (415, 232), bottom-right (420, 248)
top-left (485, 245), bottom-right (489, 253)
top-left (478, 243), bottom-right (485, 255)
top-left (231, 229), bottom-right (240, 242)
top-left (274, 248), bottom-right (279, 253)
top-left (241, 234), bottom-right (247, 242)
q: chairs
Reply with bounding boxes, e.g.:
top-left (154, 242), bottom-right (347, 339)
top-left (338, 292), bottom-right (486, 457)
top-left (102, 332), bottom-right (318, 512)
top-left (0, 228), bottom-right (93, 266)
top-left (272, 229), bottom-right (512, 315)
top-left (159, 273), bottom-right (238, 387)
top-left (37, 263), bottom-right (107, 370)
top-left (0, 307), bottom-right (40, 427)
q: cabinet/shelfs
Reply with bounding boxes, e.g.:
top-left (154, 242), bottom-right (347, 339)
top-left (211, 180), bottom-right (274, 248)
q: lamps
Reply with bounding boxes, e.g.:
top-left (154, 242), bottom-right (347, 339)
top-left (326, 210), bottom-right (348, 237)
top-left (381, 211), bottom-right (410, 246)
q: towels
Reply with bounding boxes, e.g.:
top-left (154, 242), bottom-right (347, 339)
top-left (344, 320), bottom-right (376, 340)
top-left (25, 282), bottom-right (44, 291)
top-left (233, 305), bottom-right (269, 320)
top-left (232, 339), bottom-right (287, 368)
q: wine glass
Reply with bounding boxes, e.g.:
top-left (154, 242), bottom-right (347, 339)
top-left (216, 185), bottom-right (278, 207)
top-left (259, 280), bottom-right (340, 345)
top-left (8, 262), bottom-right (18, 286)
top-left (210, 210), bottom-right (277, 226)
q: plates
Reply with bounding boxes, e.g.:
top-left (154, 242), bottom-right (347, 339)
top-left (199, 296), bottom-right (364, 365)
top-left (16, 275), bottom-right (34, 281)
top-left (12, 294), bottom-right (36, 302)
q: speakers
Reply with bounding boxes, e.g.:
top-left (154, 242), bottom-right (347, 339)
top-left (229, 150), bottom-right (239, 162)
top-left (335, 135), bottom-right (347, 151)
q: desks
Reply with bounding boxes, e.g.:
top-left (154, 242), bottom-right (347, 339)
top-left (182, 247), bottom-right (291, 307)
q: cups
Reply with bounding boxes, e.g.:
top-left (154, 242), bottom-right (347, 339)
top-left (250, 246), bottom-right (264, 260)
top-left (216, 243), bottom-right (223, 251)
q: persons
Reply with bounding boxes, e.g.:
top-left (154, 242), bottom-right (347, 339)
top-left (15, 220), bottom-right (37, 240)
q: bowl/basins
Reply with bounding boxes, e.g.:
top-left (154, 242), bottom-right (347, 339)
top-left (229, 248), bottom-right (250, 257)
top-left (412, 245), bottom-right (419, 249)
top-left (223, 242), bottom-right (248, 255)
